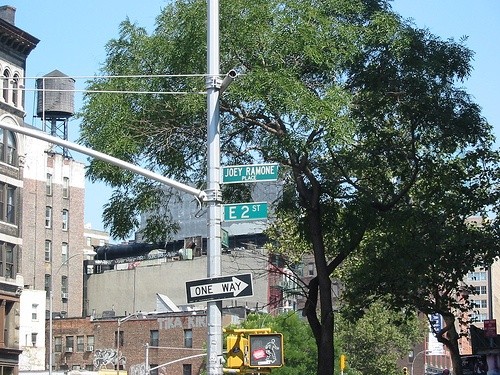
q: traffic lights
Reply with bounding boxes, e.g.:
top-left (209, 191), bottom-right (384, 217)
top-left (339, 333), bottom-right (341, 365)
top-left (403, 367), bottom-right (408, 375)
top-left (340, 355), bottom-right (347, 369)
top-left (247, 333), bottom-right (284, 368)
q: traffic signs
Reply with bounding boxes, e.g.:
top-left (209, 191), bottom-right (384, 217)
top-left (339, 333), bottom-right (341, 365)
top-left (185, 272), bottom-right (255, 305)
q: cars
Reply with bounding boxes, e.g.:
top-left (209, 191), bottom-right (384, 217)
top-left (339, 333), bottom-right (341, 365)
top-left (426, 367), bottom-right (451, 375)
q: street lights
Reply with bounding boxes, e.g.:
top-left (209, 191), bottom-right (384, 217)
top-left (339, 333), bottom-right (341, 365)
top-left (116, 311), bottom-right (148, 375)
top-left (411, 350), bottom-right (432, 375)
top-left (49, 250), bottom-right (97, 375)
